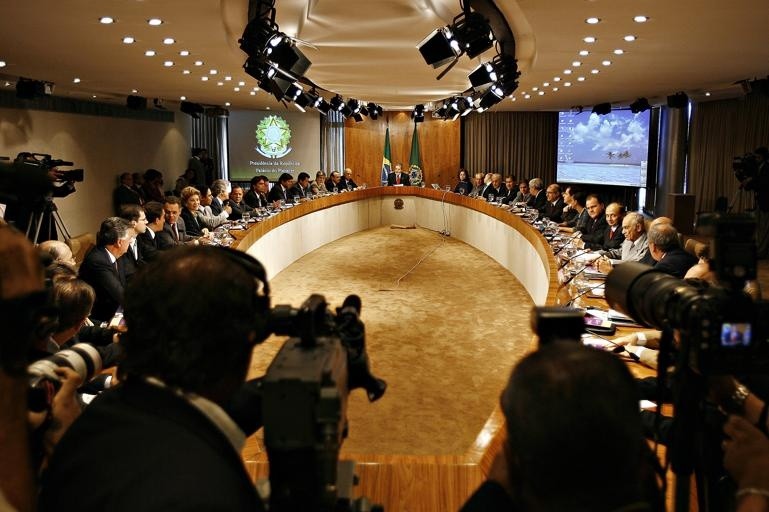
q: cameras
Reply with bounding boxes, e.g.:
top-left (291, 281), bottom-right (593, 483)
top-left (723, 152), bottom-right (758, 188)
top-left (604, 261), bottom-right (762, 381)
top-left (17, 341), bottom-right (105, 412)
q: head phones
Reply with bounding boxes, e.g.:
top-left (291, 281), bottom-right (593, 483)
top-left (126, 242), bottom-right (274, 353)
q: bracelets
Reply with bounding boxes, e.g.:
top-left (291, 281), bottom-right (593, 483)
top-left (735, 487), bottom-right (768, 499)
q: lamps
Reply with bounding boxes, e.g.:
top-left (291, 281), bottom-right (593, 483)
top-left (294, 94), bottom-right (313, 113)
top-left (330, 97), bottom-right (345, 111)
top-left (415, 12), bottom-right (492, 65)
top-left (317, 100), bottom-right (330, 115)
top-left (353, 114), bottom-right (363, 122)
top-left (375, 106), bottom-right (383, 116)
top-left (478, 90), bottom-right (502, 110)
top-left (438, 104), bottom-right (456, 118)
top-left (270, 71), bottom-right (303, 103)
top-left (360, 105), bottom-right (368, 116)
top-left (456, 96), bottom-right (473, 117)
top-left (238, 18), bottom-right (282, 56)
top-left (340, 105), bottom-right (351, 117)
top-left (242, 55), bottom-right (277, 82)
top-left (414, 104), bottom-right (425, 122)
top-left (467, 63), bottom-right (492, 88)
top-left (272, 41), bottom-right (312, 76)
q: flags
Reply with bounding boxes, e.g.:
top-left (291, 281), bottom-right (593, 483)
top-left (381, 127), bottom-right (392, 186)
top-left (409, 122), bottom-right (423, 186)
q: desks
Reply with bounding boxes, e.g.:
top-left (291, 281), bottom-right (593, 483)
top-left (116, 186), bottom-right (415, 512)
top-left (416, 186), bottom-right (708, 510)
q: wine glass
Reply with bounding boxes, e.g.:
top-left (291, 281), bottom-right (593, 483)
top-left (223, 222), bottom-right (232, 239)
top-left (488, 193), bottom-right (494, 202)
top-left (460, 188), bottom-right (465, 195)
top-left (530, 209), bottom-right (539, 222)
top-left (267, 203), bottom-right (274, 214)
top-left (496, 197), bottom-right (502, 206)
top-left (348, 185), bottom-right (352, 192)
top-left (242, 213), bottom-right (251, 228)
top-left (293, 196), bottom-right (300, 205)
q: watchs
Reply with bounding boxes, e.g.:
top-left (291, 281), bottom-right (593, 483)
top-left (728, 384), bottom-right (749, 409)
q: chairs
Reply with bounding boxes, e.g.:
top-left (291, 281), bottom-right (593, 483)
top-left (683, 238), bottom-right (709, 260)
top-left (65, 232), bottom-right (96, 268)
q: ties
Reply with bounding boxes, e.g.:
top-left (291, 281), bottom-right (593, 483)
top-left (610, 227), bottom-right (614, 239)
top-left (171, 222), bottom-right (180, 242)
top-left (396, 174), bottom-right (401, 185)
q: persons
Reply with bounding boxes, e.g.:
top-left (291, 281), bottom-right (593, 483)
top-left (387, 163), bottom-right (411, 186)
top-left (608, 245), bottom-right (710, 374)
top-left (113, 172), bottom-right (142, 215)
top-left (138, 169), bottom-right (161, 202)
top-left (468, 173), bottom-right (485, 198)
top-left (260, 175), bottom-right (273, 206)
top-left (509, 178), bottom-right (532, 207)
top-left (574, 202), bottom-right (626, 251)
top-left (634, 370), bottom-right (676, 445)
top-left (724, 324), bottom-right (743, 342)
top-left (196, 185), bottom-right (233, 228)
top-left (33, 244), bottom-right (273, 511)
top-left (211, 179), bottom-right (230, 220)
top-left (526, 178), bottom-right (547, 209)
top-left (487, 173), bottom-right (506, 203)
top-left (38, 240), bottom-right (77, 269)
top-left (499, 175), bottom-right (519, 204)
top-left (535, 183), bottom-right (564, 223)
top-left (156, 195), bottom-right (212, 251)
top-left (647, 223), bottom-right (699, 280)
top-left (3, 151), bottom-right (76, 244)
top-left (454, 169), bottom-right (473, 195)
top-left (46, 279), bottom-right (121, 368)
top-left (479, 173), bottom-right (494, 198)
top-left (0, 219), bottom-right (81, 512)
top-left (78, 216), bottom-right (131, 323)
top-left (137, 201), bottom-right (166, 262)
top-left (130, 172), bottom-right (142, 193)
top-left (720, 375), bottom-right (769, 512)
top-left (179, 186), bottom-right (210, 238)
top-left (289, 172), bottom-right (310, 198)
top-left (340, 168), bottom-right (358, 191)
top-left (556, 193), bottom-right (589, 233)
top-left (562, 184), bottom-right (585, 222)
top-left (454, 345), bottom-right (668, 512)
top-left (310, 170), bottom-right (328, 194)
top-left (638, 217), bottom-right (673, 267)
top-left (223, 186), bottom-right (258, 221)
top-left (326, 171), bottom-right (343, 193)
top-left (576, 194), bottom-right (608, 243)
top-left (599, 213), bottom-right (649, 264)
top-left (243, 176), bottom-right (267, 214)
top-left (45, 263), bottom-right (127, 345)
top-left (266, 173), bottom-right (294, 204)
top-left (118, 204), bottom-right (158, 281)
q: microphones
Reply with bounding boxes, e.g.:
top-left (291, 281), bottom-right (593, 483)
top-left (551, 250), bottom-right (589, 271)
top-left (228, 218), bottom-right (248, 231)
top-left (216, 221), bottom-right (238, 240)
top-left (559, 248), bottom-right (620, 290)
top-left (563, 282), bottom-right (607, 306)
top-left (180, 228), bottom-right (227, 248)
top-left (552, 232), bottom-right (581, 255)
